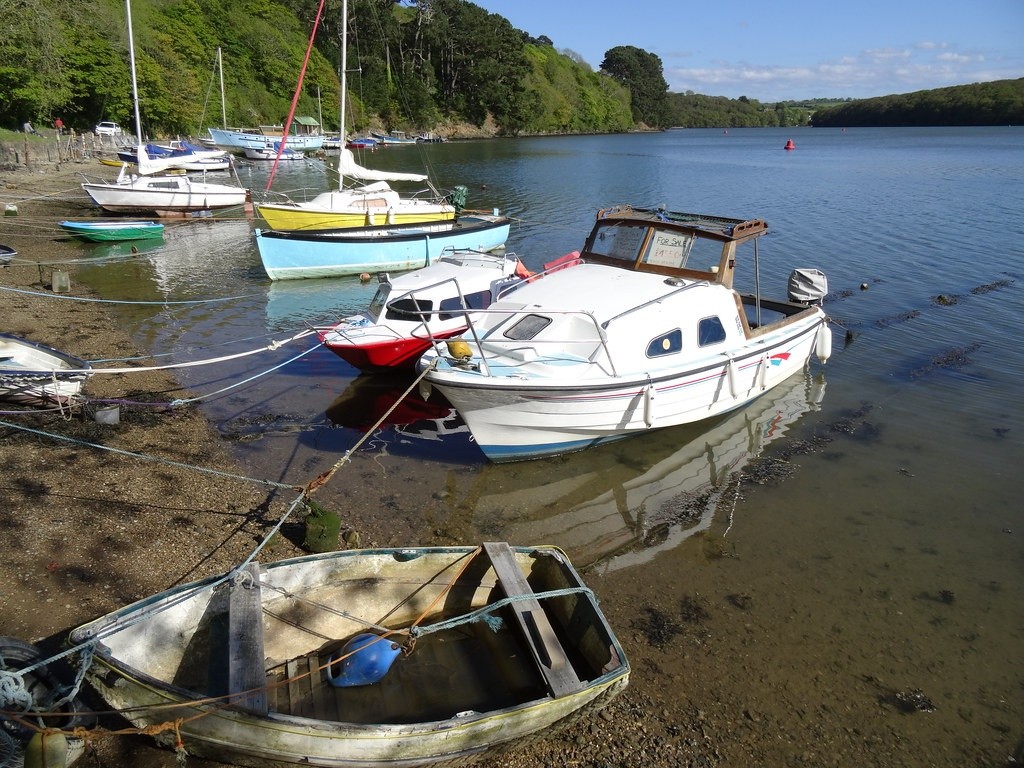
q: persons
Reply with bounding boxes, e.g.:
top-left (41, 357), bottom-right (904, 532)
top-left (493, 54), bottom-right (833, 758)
top-left (24, 121), bottom-right (37, 133)
top-left (55, 118), bottom-right (62, 135)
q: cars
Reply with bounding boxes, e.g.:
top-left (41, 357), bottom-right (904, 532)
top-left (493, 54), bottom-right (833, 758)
top-left (94, 120), bottom-right (121, 137)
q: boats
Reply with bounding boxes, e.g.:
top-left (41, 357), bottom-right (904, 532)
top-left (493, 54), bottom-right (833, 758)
top-left (0, 244), bottom-right (18, 266)
top-left (314, 250), bottom-right (543, 375)
top-left (415, 202), bottom-right (836, 461)
top-left (96, 46), bottom-right (447, 174)
top-left (65, 540), bottom-right (633, 768)
top-left (255, 206), bottom-right (512, 282)
top-left (0, 333), bottom-right (96, 411)
top-left (56, 217), bottom-right (165, 242)
top-left (76, 1), bottom-right (249, 213)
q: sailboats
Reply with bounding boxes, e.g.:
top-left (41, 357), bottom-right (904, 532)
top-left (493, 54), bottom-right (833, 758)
top-left (252, 1), bottom-right (461, 233)
